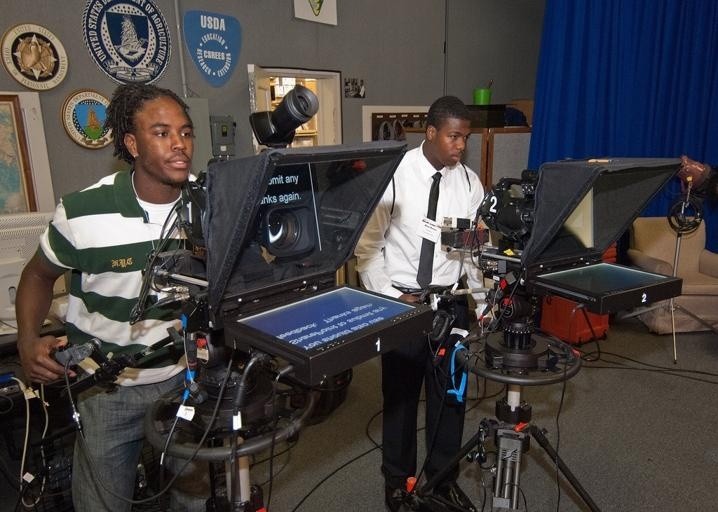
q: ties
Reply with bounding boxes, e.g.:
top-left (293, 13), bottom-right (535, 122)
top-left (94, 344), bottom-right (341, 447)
top-left (417, 171), bottom-right (442, 288)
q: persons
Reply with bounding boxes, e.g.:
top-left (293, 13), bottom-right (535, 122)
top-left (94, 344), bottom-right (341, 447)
top-left (15, 82), bottom-right (206, 511)
top-left (355, 96), bottom-right (495, 512)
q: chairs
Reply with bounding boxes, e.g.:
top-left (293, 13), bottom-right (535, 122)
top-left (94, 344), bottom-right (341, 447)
top-left (627, 214), bottom-right (717, 335)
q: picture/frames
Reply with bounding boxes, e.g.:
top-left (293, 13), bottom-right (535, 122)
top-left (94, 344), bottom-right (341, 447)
top-left (0, 91), bottom-right (57, 216)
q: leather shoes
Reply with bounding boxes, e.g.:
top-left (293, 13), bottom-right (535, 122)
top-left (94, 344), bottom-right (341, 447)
top-left (385, 481), bottom-right (417, 511)
top-left (434, 481), bottom-right (476, 511)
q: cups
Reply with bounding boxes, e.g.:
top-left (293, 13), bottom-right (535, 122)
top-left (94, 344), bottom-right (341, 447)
top-left (473, 88), bottom-right (491, 106)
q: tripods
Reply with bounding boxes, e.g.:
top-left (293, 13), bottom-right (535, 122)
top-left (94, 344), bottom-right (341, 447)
top-left (392, 384), bottom-right (602, 512)
